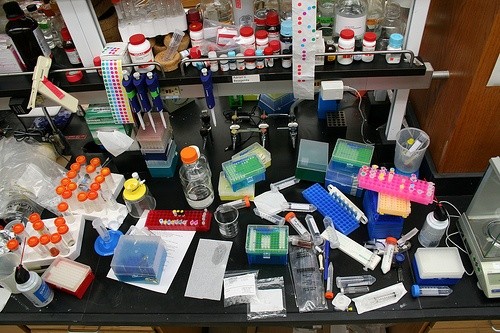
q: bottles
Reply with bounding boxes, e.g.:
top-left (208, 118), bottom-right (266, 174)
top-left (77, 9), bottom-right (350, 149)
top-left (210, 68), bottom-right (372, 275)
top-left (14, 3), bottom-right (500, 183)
top-left (417, 205), bottom-right (448, 247)
top-left (187, 1), bottom-right (405, 71)
top-left (177, 144), bottom-right (214, 209)
top-left (128, 35), bottom-right (157, 75)
top-left (15, 265), bottom-right (54, 307)
top-left (123, 179), bottom-right (157, 217)
top-left (1, 0), bottom-right (80, 73)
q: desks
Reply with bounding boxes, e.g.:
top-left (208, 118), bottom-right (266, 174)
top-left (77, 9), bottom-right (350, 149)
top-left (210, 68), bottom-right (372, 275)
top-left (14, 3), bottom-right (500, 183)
top-left (1, 147), bottom-right (500, 332)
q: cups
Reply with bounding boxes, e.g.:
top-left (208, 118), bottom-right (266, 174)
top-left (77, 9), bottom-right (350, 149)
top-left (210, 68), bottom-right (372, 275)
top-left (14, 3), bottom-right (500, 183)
top-left (0, 254), bottom-right (31, 295)
top-left (216, 205), bottom-right (239, 238)
top-left (393, 128), bottom-right (429, 174)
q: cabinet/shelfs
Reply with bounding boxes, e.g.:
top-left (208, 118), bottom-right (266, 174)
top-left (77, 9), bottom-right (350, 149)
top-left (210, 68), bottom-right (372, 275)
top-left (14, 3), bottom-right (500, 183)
top-left (0, 0), bottom-right (435, 145)
